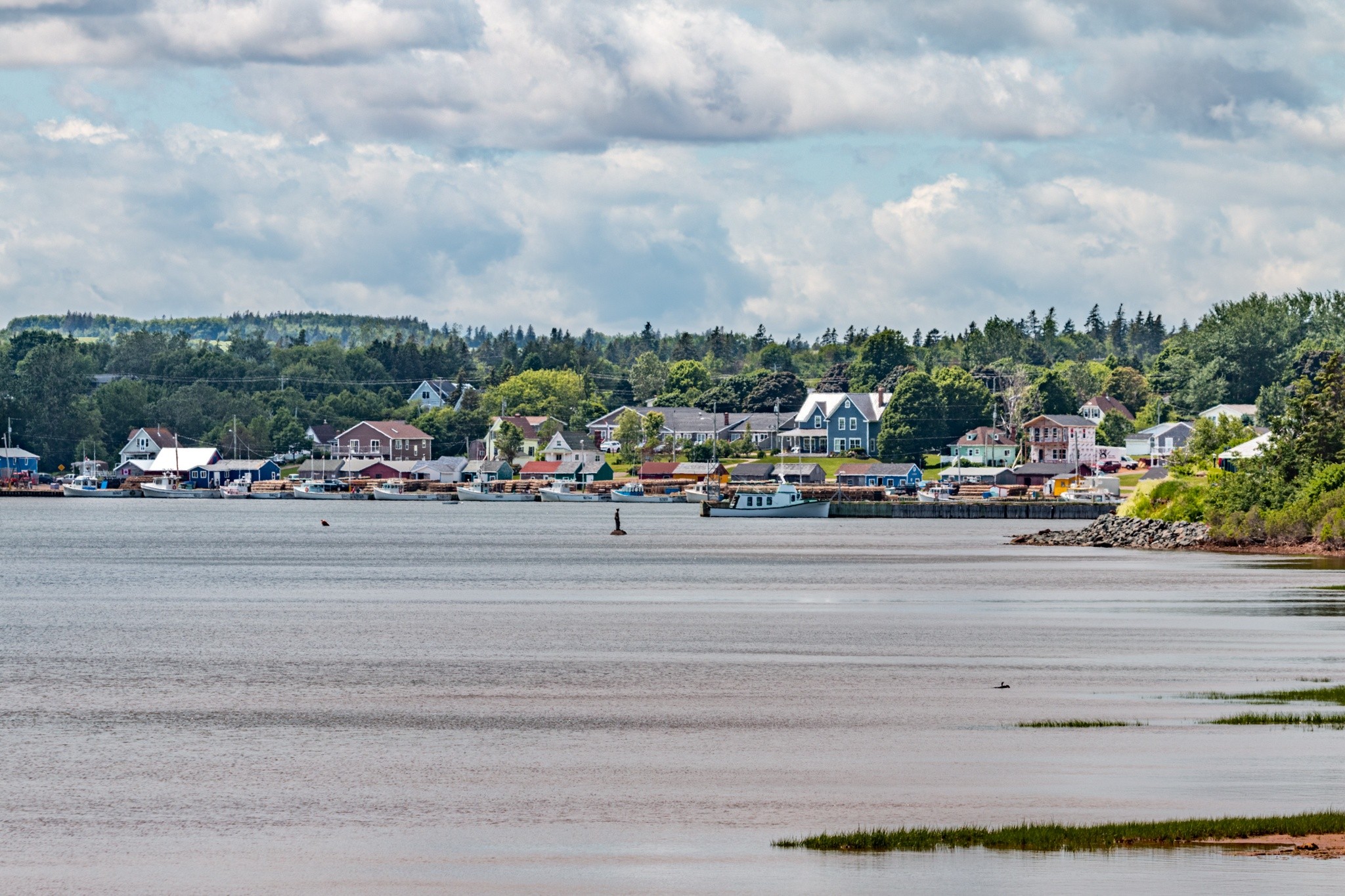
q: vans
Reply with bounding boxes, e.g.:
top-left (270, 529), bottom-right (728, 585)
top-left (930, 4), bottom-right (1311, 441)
top-left (600, 441), bottom-right (622, 453)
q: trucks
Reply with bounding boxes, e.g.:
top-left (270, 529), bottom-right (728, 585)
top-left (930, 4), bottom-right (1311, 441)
top-left (1097, 446), bottom-right (1138, 469)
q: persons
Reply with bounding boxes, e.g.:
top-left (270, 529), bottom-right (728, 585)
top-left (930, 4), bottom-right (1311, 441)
top-left (210, 478), bottom-right (219, 491)
top-left (511, 482), bottom-right (532, 494)
top-left (352, 486), bottom-right (361, 493)
top-left (615, 509), bottom-right (620, 530)
top-left (916, 485), bottom-right (919, 491)
top-left (811, 488), bottom-right (826, 499)
top-left (280, 482), bottom-right (287, 490)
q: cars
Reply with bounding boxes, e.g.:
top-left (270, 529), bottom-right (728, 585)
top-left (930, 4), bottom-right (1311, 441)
top-left (56, 473), bottom-right (78, 485)
top-left (656, 444), bottom-right (672, 454)
top-left (38, 472), bottom-right (55, 484)
top-left (1096, 459), bottom-right (1121, 473)
top-left (2, 472), bottom-right (30, 487)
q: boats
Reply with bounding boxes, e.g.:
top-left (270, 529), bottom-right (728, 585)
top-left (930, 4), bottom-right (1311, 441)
top-left (373, 482), bottom-right (453, 501)
top-left (610, 482), bottom-right (675, 503)
top-left (538, 481), bottom-right (599, 503)
top-left (708, 482), bottom-right (831, 518)
top-left (220, 477), bottom-right (285, 499)
top-left (685, 482), bottom-right (723, 501)
top-left (140, 474), bottom-right (221, 498)
top-left (457, 478), bottom-right (536, 502)
top-left (62, 475), bottom-right (142, 497)
top-left (292, 485), bottom-right (369, 500)
